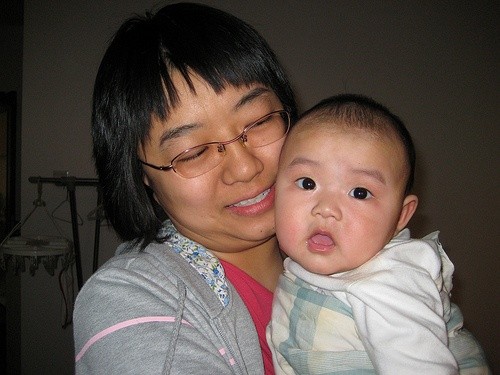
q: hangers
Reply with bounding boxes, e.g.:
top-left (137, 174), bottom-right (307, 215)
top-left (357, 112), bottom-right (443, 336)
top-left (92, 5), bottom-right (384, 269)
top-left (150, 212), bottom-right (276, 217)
top-left (50, 186), bottom-right (85, 227)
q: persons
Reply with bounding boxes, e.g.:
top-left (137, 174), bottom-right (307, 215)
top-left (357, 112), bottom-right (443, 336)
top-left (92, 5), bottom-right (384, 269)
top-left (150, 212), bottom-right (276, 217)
top-left (75, 1), bottom-right (299, 374)
top-left (259, 94), bottom-right (491, 374)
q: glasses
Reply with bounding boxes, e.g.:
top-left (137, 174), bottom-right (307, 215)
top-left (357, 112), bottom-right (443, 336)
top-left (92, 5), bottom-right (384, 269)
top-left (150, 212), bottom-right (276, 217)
top-left (137, 105), bottom-right (293, 180)
